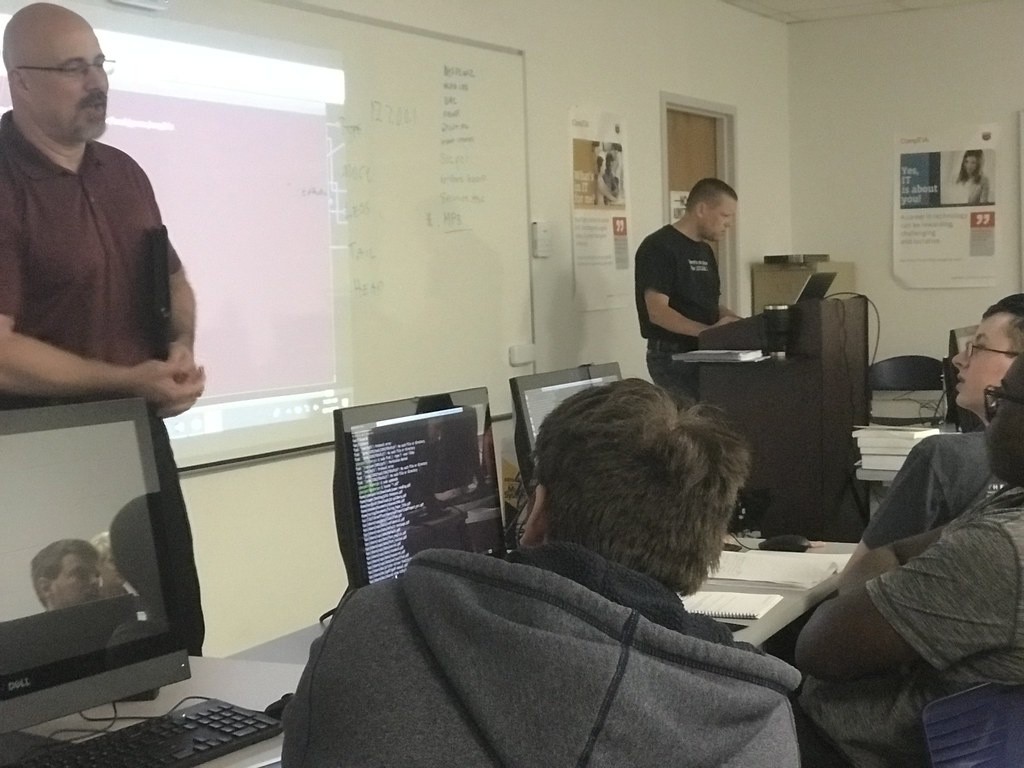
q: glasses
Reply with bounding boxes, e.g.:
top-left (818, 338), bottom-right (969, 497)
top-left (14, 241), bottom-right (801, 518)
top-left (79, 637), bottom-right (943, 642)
top-left (18, 61), bottom-right (118, 78)
top-left (966, 341), bottom-right (1020, 358)
top-left (984, 385), bottom-right (1024, 424)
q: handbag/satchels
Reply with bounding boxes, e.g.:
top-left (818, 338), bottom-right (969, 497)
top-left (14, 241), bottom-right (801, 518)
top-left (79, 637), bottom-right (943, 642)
top-left (148, 226), bottom-right (171, 362)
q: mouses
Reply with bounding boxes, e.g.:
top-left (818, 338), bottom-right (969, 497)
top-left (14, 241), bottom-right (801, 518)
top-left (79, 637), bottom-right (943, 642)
top-left (758, 535), bottom-right (811, 553)
top-left (265, 692), bottom-right (295, 720)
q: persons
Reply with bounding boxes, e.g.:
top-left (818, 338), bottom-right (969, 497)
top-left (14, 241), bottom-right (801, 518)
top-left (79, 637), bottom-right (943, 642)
top-left (0, 5), bottom-right (206, 660)
top-left (634, 176), bottom-right (746, 405)
top-left (761, 294), bottom-right (1024, 768)
top-left (282, 378), bottom-right (805, 767)
top-left (589, 142), bottom-right (624, 209)
top-left (413, 393), bottom-right (493, 519)
top-left (29, 491), bottom-right (169, 618)
top-left (948, 148), bottom-right (990, 203)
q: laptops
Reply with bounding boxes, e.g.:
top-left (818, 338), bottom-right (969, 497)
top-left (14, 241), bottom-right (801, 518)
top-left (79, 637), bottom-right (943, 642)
top-left (795, 271), bottom-right (837, 306)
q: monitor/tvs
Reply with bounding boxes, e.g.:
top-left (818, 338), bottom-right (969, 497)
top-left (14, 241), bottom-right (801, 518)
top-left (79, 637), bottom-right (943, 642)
top-left (509, 361), bottom-right (626, 499)
top-left (0, 395), bottom-right (193, 768)
top-left (330, 386), bottom-right (507, 589)
top-left (943, 323), bottom-right (983, 433)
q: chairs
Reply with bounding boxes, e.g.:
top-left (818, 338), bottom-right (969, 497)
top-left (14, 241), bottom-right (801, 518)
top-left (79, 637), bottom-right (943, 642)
top-left (864, 355), bottom-right (949, 426)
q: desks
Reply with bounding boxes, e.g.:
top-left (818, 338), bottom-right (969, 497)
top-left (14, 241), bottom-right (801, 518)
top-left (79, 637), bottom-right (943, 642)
top-left (752, 260), bottom-right (854, 315)
top-left (214, 537), bottom-right (858, 768)
top-left (856, 467), bottom-right (898, 520)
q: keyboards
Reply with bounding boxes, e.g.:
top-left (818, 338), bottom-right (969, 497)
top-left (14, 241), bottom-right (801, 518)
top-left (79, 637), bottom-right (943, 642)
top-left (5, 700), bottom-right (281, 768)
